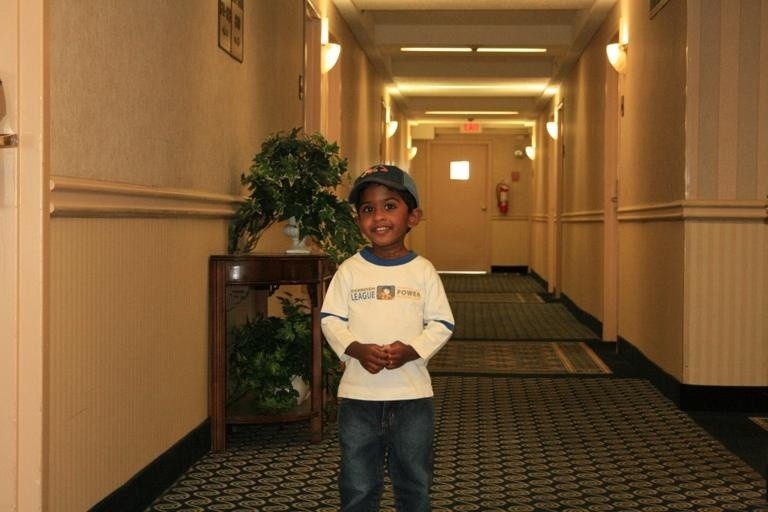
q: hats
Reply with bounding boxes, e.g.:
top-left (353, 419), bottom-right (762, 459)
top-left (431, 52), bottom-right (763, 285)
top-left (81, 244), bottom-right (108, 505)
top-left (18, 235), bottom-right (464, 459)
top-left (348, 164), bottom-right (420, 208)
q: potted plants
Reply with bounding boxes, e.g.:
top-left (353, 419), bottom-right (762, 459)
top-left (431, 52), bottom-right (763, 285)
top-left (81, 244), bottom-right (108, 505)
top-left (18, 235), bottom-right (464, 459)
top-left (226, 125), bottom-right (370, 266)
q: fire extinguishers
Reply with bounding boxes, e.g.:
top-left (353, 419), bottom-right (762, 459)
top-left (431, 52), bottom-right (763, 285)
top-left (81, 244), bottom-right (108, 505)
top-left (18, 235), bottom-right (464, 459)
top-left (496, 179), bottom-right (510, 214)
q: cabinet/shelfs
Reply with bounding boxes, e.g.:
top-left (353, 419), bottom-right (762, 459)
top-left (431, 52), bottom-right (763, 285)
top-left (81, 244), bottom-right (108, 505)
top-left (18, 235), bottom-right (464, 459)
top-left (209, 250), bottom-right (344, 452)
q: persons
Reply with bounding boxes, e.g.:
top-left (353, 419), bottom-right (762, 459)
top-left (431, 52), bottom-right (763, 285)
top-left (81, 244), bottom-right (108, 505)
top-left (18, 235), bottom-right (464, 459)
top-left (321, 168), bottom-right (455, 512)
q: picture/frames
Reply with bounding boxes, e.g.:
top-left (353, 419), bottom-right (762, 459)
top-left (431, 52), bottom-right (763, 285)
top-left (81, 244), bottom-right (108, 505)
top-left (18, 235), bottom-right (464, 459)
top-left (218, 0), bottom-right (245, 63)
top-left (646, 0), bottom-right (671, 19)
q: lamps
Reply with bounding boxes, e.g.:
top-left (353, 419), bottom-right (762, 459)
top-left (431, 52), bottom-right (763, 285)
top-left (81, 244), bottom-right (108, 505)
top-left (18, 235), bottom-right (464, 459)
top-left (525, 145), bottom-right (535, 161)
top-left (384, 120), bottom-right (399, 137)
top-left (605, 42), bottom-right (629, 73)
top-left (406, 146), bottom-right (418, 160)
top-left (320, 43), bottom-right (342, 74)
top-left (546, 121), bottom-right (558, 140)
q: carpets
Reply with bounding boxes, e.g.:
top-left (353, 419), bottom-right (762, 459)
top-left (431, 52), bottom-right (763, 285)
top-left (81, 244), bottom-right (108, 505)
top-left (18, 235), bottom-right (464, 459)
top-left (142, 274), bottom-right (767, 512)
